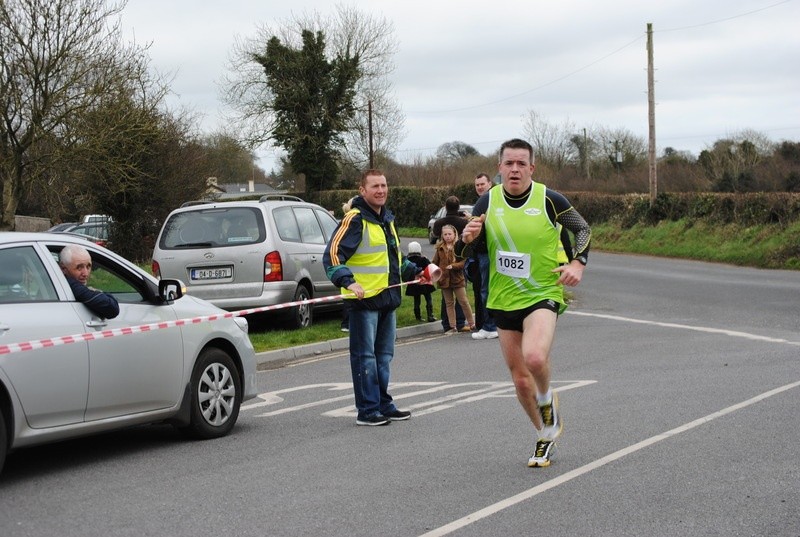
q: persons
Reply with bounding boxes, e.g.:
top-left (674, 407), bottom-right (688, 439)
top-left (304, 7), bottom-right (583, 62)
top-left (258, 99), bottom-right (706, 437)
top-left (341, 195), bottom-right (359, 332)
top-left (462, 138), bottom-right (592, 466)
top-left (401, 173), bottom-right (502, 338)
top-left (56, 245), bottom-right (119, 320)
top-left (322, 170), bottom-right (428, 425)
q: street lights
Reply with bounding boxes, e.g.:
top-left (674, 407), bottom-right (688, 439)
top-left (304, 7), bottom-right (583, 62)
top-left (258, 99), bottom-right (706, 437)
top-left (345, 87), bottom-right (375, 171)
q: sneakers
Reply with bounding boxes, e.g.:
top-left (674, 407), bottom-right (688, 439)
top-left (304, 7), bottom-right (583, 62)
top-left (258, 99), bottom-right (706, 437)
top-left (537, 387), bottom-right (563, 441)
top-left (527, 437), bottom-right (557, 467)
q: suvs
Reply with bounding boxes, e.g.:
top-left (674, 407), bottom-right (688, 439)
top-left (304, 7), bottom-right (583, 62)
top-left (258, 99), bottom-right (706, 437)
top-left (150, 181), bottom-right (347, 331)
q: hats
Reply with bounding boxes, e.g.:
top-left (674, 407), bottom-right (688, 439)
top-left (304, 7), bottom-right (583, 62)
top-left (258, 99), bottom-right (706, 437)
top-left (409, 242), bottom-right (421, 254)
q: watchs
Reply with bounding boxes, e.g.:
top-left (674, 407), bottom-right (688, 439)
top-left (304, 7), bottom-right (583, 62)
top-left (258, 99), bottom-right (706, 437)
top-left (575, 255), bottom-right (587, 265)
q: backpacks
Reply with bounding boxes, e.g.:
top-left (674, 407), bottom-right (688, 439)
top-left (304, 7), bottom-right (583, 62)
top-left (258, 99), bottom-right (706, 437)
top-left (401, 255), bottom-right (418, 283)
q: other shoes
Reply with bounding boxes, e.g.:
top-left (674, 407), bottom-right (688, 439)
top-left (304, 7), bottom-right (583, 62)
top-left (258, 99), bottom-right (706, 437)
top-left (416, 319), bottom-right (425, 322)
top-left (356, 412), bottom-right (392, 426)
top-left (471, 329), bottom-right (499, 339)
top-left (340, 322), bottom-right (349, 332)
top-left (427, 317), bottom-right (436, 322)
top-left (460, 325), bottom-right (470, 331)
top-left (469, 326), bottom-right (479, 333)
top-left (380, 410), bottom-right (411, 420)
top-left (444, 328), bottom-right (458, 335)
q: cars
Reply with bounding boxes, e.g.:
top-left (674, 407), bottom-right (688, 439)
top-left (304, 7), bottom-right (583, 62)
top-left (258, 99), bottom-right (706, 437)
top-left (0, 227), bottom-right (261, 481)
top-left (46, 214), bottom-right (124, 249)
top-left (427, 204), bottom-right (476, 245)
